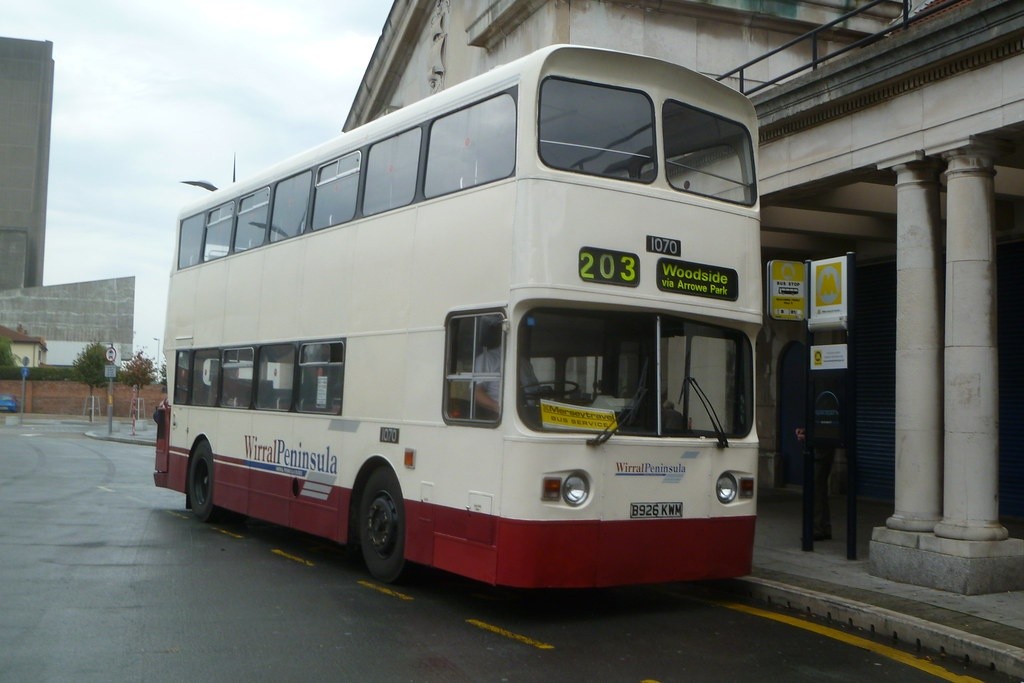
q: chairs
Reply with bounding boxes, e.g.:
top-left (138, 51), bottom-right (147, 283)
top-left (233, 395), bottom-right (314, 411)
top-left (206, 176), bottom-right (473, 259)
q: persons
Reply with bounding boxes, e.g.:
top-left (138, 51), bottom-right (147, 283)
top-left (796, 427), bottom-right (835, 541)
top-left (663, 400), bottom-right (683, 428)
top-left (469, 323), bottom-right (541, 415)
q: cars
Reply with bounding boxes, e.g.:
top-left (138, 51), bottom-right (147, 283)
top-left (0, 393), bottom-right (18, 413)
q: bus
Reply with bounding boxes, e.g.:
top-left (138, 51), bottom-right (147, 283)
top-left (152, 42), bottom-right (766, 594)
